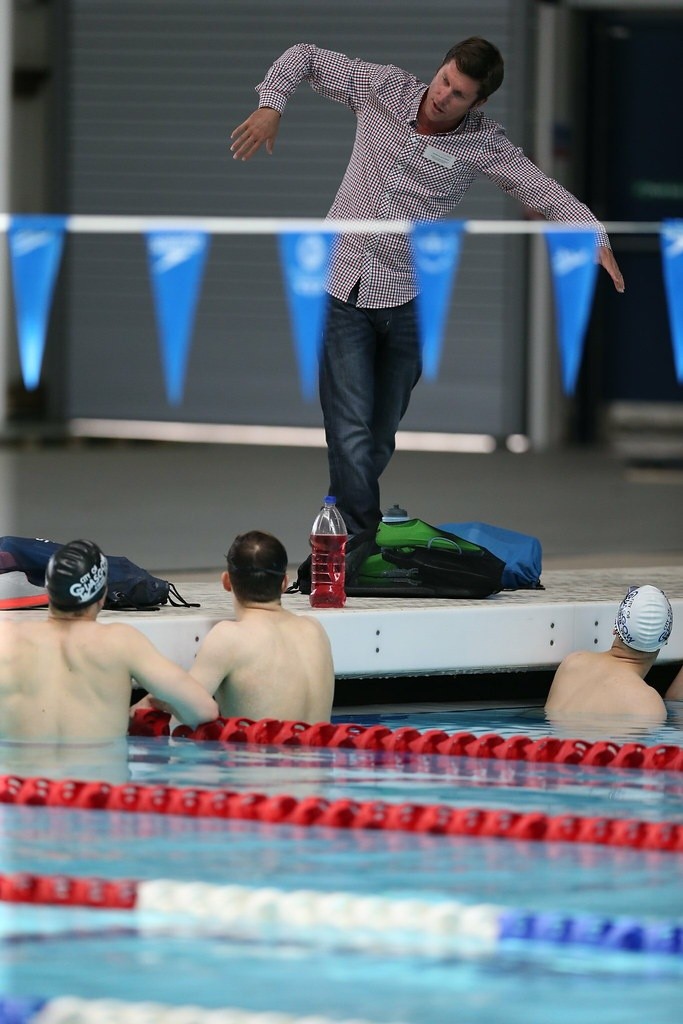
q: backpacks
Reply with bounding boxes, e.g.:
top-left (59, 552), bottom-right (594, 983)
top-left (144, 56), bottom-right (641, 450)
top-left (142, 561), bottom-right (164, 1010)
top-left (285, 519), bottom-right (506, 600)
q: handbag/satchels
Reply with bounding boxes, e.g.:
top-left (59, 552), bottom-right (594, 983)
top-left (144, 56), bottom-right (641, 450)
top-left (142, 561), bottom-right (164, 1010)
top-left (0, 535), bottom-right (200, 610)
top-left (433, 521), bottom-right (546, 590)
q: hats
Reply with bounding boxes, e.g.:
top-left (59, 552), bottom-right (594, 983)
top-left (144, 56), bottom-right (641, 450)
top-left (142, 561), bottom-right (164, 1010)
top-left (615, 585), bottom-right (672, 652)
top-left (45, 539), bottom-right (108, 606)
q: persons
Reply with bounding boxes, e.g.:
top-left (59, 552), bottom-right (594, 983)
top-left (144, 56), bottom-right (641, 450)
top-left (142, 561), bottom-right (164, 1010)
top-left (0, 537), bottom-right (221, 769)
top-left (129, 529), bottom-right (335, 733)
top-left (544, 586), bottom-right (672, 723)
top-left (230, 36), bottom-right (626, 597)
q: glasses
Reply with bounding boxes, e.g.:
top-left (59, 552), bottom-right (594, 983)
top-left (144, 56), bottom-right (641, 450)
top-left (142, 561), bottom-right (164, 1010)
top-left (628, 586), bottom-right (664, 595)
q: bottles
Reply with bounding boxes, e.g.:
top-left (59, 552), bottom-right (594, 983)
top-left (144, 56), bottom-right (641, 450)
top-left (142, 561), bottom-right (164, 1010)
top-left (307, 494), bottom-right (349, 608)
